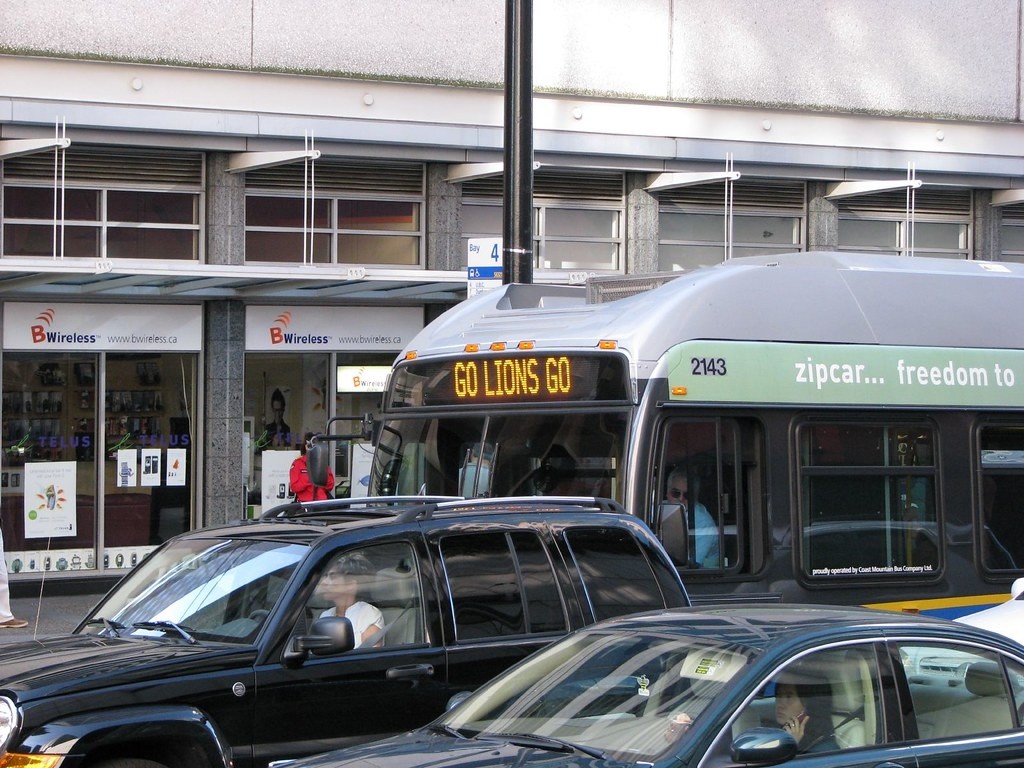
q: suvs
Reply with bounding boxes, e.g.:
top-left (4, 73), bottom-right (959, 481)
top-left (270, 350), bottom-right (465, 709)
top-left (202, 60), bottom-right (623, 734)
top-left (0, 495), bottom-right (694, 767)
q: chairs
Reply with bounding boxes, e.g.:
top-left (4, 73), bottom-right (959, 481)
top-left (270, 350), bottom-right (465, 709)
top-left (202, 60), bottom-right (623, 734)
top-left (782, 647), bottom-right (869, 748)
top-left (367, 565), bottom-right (427, 648)
top-left (668, 646), bottom-right (750, 724)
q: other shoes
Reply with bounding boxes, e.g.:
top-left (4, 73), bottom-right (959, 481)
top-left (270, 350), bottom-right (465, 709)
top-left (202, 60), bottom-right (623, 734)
top-left (0, 619), bottom-right (28, 628)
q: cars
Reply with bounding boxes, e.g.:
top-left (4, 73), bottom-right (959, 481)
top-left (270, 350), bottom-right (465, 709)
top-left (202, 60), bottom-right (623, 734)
top-left (955, 575), bottom-right (1024, 643)
top-left (268, 602), bottom-right (1024, 767)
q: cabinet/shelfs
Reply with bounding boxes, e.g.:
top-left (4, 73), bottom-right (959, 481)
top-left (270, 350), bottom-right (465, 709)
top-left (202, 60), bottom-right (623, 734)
top-left (2, 358), bottom-right (171, 471)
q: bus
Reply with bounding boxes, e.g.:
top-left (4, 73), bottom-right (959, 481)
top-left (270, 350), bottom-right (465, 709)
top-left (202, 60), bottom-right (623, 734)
top-left (304, 253), bottom-right (1023, 620)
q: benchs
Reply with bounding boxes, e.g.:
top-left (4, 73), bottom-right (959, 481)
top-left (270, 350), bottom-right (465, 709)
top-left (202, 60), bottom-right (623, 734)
top-left (896, 647), bottom-right (1017, 739)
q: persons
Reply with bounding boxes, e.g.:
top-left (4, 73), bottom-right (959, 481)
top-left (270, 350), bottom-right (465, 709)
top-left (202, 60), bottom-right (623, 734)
top-left (541, 444), bottom-right (600, 506)
top-left (418, 442), bottom-right (494, 504)
top-left (657, 465), bottom-right (723, 570)
top-left (308, 554), bottom-right (385, 654)
top-left (290, 431), bottom-right (335, 503)
top-left (675, 664), bottom-right (841, 754)
top-left (265, 388), bottom-right (291, 448)
top-left (0, 528), bottom-right (29, 629)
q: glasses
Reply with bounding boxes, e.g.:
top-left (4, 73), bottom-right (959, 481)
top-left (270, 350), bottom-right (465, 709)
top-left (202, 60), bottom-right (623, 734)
top-left (669, 487), bottom-right (689, 498)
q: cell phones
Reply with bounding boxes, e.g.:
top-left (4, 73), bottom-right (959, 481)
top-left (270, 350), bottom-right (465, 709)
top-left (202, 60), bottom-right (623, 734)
top-left (45, 558), bottom-right (50, 570)
top-left (2, 473), bottom-right (20, 486)
top-left (118, 555), bottom-right (122, 566)
top-left (145, 456), bottom-right (158, 473)
top-left (279, 483), bottom-right (285, 498)
top-left (289, 485), bottom-right (295, 498)
top-left (104, 555), bottom-right (108, 566)
top-left (131, 554), bottom-right (136, 566)
